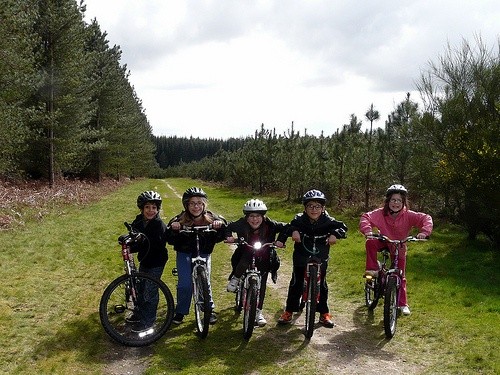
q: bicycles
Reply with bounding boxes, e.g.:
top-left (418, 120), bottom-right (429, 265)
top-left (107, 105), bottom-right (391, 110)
top-left (291, 232), bottom-right (340, 337)
top-left (363, 232), bottom-right (428, 338)
top-left (224, 237), bottom-right (286, 339)
top-left (169, 224), bottom-right (221, 338)
top-left (99, 222), bottom-right (174, 346)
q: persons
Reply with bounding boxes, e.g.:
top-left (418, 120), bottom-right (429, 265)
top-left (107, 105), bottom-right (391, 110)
top-left (224, 199), bottom-right (290, 326)
top-left (278, 190), bottom-right (348, 327)
top-left (359, 184), bottom-right (433, 316)
top-left (165, 186), bottom-right (227, 324)
top-left (126, 191), bottom-right (169, 333)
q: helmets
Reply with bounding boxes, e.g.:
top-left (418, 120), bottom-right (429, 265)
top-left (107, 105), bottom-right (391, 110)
top-left (303, 189), bottom-right (326, 200)
top-left (386, 184), bottom-right (408, 195)
top-left (243, 198), bottom-right (267, 215)
top-left (182, 187), bottom-right (207, 200)
top-left (137, 190), bottom-right (162, 209)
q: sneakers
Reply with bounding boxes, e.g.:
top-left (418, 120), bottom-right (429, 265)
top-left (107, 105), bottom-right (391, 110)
top-left (278, 312), bottom-right (292, 323)
top-left (319, 313), bottom-right (334, 327)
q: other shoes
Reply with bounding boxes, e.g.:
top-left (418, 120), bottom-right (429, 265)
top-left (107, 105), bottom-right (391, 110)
top-left (255, 313), bottom-right (267, 326)
top-left (365, 270), bottom-right (378, 279)
top-left (131, 321), bottom-right (158, 333)
top-left (210, 308), bottom-right (218, 324)
top-left (173, 313), bottom-right (184, 325)
top-left (126, 314), bottom-right (140, 322)
top-left (227, 276), bottom-right (240, 293)
top-left (398, 306), bottom-right (410, 315)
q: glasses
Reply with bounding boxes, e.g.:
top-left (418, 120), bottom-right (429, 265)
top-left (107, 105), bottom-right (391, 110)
top-left (189, 201), bottom-right (203, 207)
top-left (390, 197), bottom-right (402, 204)
top-left (249, 214), bottom-right (261, 219)
top-left (306, 204), bottom-right (323, 210)
